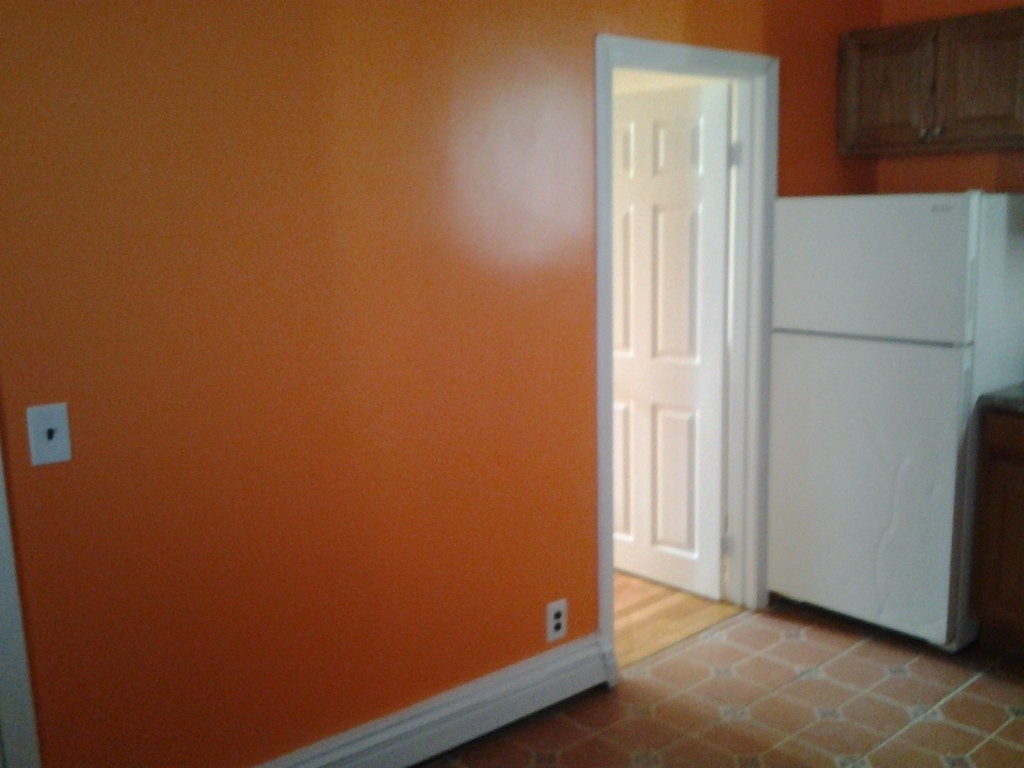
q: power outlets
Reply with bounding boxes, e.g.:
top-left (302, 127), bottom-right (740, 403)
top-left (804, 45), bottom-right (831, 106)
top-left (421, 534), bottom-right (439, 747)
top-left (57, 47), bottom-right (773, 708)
top-left (547, 599), bottom-right (567, 642)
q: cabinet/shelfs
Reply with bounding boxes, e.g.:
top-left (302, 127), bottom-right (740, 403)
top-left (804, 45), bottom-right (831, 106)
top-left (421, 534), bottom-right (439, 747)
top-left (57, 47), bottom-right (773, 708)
top-left (970, 409), bottom-right (1024, 664)
top-left (838, 6), bottom-right (1024, 155)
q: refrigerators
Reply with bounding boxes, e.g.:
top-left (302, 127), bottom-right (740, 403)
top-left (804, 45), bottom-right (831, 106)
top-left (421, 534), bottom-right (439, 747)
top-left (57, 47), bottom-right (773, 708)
top-left (771, 193), bottom-right (1024, 651)
